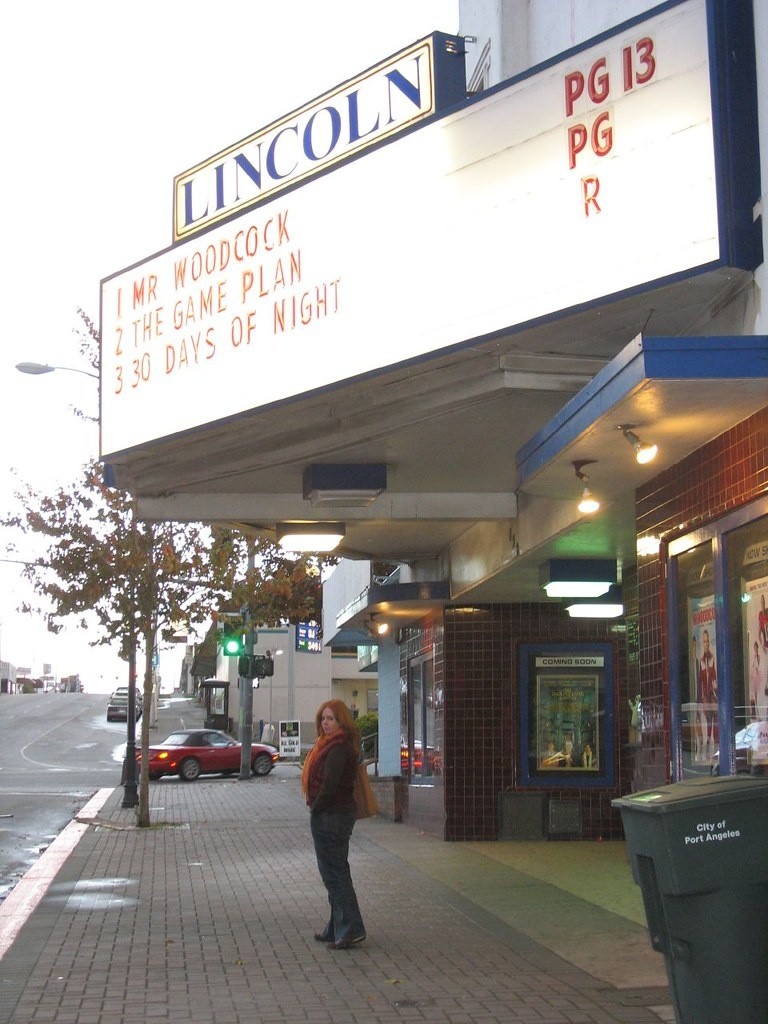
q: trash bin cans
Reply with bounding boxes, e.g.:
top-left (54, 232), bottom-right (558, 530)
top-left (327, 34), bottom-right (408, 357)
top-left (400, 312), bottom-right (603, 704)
top-left (612, 778), bottom-right (768, 1024)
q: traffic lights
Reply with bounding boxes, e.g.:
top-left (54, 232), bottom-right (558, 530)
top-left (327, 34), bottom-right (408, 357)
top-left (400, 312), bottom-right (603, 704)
top-left (222, 637), bottom-right (243, 657)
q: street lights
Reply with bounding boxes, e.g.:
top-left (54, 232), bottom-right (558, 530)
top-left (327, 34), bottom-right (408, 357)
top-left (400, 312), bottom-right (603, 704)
top-left (15, 360), bottom-right (138, 810)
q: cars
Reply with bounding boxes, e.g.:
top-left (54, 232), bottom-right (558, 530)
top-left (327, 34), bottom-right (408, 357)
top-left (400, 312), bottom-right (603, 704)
top-left (134, 728), bottom-right (278, 782)
top-left (107, 686), bottom-right (143, 722)
top-left (400, 738), bottom-right (436, 776)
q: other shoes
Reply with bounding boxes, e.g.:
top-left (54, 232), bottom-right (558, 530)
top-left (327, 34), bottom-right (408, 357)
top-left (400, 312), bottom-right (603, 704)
top-left (315, 932), bottom-right (335, 941)
top-left (329, 933), bottom-right (366, 948)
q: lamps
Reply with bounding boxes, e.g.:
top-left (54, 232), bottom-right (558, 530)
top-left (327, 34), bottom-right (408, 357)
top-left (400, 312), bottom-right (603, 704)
top-left (573, 459), bottom-right (601, 513)
top-left (617, 424), bottom-right (657, 464)
top-left (302, 463), bottom-right (386, 507)
top-left (539, 557), bottom-right (618, 598)
top-left (562, 584), bottom-right (623, 620)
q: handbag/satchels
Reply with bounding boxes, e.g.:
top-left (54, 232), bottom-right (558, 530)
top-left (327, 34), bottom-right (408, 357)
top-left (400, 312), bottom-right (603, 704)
top-left (352, 763), bottom-right (378, 820)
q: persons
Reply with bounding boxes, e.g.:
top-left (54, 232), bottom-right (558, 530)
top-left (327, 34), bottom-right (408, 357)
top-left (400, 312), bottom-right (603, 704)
top-left (750, 595), bottom-right (768, 719)
top-left (302, 699), bottom-right (378, 946)
top-left (692, 631), bottom-right (718, 722)
